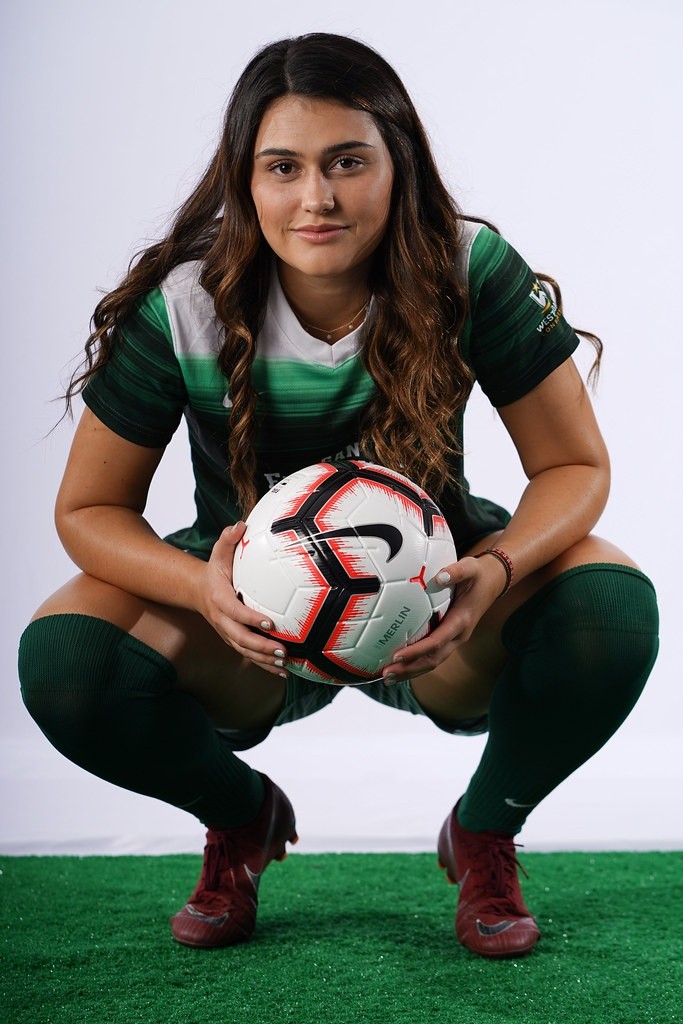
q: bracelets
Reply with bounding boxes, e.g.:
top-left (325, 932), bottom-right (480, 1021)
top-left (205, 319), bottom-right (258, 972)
top-left (475, 549), bottom-right (515, 600)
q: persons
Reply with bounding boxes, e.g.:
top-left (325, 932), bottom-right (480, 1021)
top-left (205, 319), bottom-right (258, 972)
top-left (19, 31), bottom-right (662, 958)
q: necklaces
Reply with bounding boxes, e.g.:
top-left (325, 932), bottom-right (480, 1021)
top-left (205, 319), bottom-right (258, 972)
top-left (290, 300), bottom-right (369, 339)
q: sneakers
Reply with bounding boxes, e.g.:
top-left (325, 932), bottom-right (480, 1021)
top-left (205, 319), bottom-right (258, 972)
top-left (436, 795), bottom-right (541, 954)
top-left (170, 769), bottom-right (299, 948)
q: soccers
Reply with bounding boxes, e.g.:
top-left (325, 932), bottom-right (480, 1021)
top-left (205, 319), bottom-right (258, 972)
top-left (227, 460), bottom-right (459, 685)
